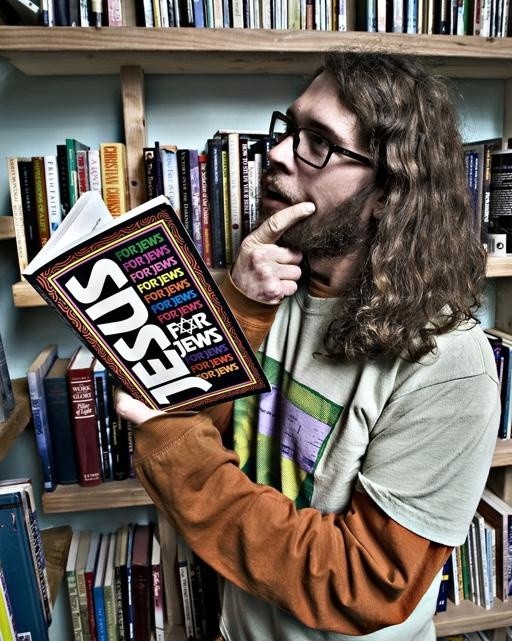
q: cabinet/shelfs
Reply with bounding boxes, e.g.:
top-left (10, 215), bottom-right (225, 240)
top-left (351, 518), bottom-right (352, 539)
top-left (0, 0), bottom-right (512, 639)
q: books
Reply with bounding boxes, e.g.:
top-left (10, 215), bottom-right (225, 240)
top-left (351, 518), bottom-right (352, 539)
top-left (1, 0), bottom-right (512, 43)
top-left (4, 129), bottom-right (287, 641)
top-left (430, 135), bottom-right (512, 641)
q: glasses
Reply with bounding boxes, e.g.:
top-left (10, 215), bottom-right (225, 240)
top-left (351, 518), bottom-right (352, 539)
top-left (266, 107), bottom-right (376, 173)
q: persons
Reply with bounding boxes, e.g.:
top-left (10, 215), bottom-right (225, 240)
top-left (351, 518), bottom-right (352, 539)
top-left (108, 48), bottom-right (503, 641)
